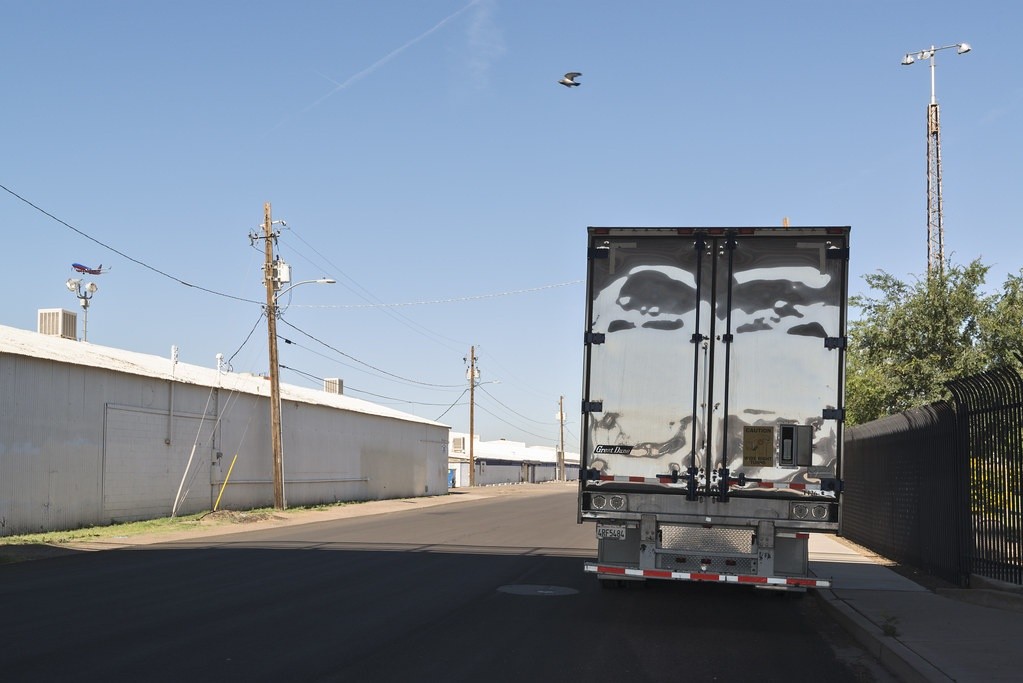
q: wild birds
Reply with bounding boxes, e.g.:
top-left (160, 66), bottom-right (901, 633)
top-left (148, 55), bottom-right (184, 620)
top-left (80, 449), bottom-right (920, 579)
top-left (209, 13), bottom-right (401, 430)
top-left (556, 71), bottom-right (583, 89)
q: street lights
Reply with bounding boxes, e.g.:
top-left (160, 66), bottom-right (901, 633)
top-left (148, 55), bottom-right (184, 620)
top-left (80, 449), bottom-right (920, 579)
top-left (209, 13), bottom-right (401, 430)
top-left (470, 381), bottom-right (502, 486)
top-left (266, 279), bottom-right (336, 511)
top-left (66, 279), bottom-right (98, 342)
top-left (560, 422), bottom-right (579, 480)
top-left (902, 42), bottom-right (973, 305)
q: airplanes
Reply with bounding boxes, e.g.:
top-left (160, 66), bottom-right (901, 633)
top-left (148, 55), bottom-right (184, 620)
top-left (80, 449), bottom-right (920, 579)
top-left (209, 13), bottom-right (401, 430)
top-left (71, 263), bottom-right (112, 275)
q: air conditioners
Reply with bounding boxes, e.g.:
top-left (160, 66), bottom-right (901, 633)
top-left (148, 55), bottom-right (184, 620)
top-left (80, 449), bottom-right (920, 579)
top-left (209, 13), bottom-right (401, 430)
top-left (323, 377), bottom-right (343, 394)
top-left (36, 308), bottom-right (79, 341)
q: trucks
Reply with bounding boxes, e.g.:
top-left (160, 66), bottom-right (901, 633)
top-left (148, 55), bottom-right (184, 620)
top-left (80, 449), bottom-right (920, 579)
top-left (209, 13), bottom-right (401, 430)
top-left (577, 225), bottom-right (851, 595)
